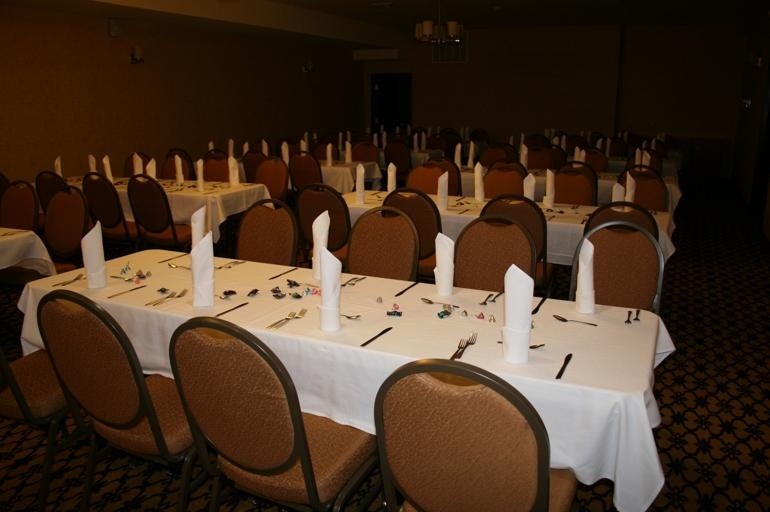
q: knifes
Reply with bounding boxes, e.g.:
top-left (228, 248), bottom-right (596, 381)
top-left (554, 353), bottom-right (572, 378)
top-left (107, 284), bottom-right (146, 298)
top-left (532, 291), bottom-right (549, 314)
top-left (269, 268), bottom-right (298, 279)
top-left (395, 281), bottom-right (419, 296)
top-left (158, 251), bottom-right (189, 263)
top-left (360, 327), bottom-right (392, 346)
top-left (215, 302), bottom-right (248, 316)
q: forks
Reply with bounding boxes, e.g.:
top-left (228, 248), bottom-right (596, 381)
top-left (266, 307), bottom-right (307, 328)
top-left (449, 331), bottom-right (477, 360)
top-left (624, 309), bottom-right (640, 327)
top-left (51, 272), bottom-right (87, 287)
top-left (145, 288), bottom-right (188, 306)
top-left (478, 290), bottom-right (503, 305)
top-left (342, 277), bottom-right (366, 286)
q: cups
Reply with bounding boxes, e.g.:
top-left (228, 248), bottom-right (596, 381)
top-left (84, 264), bottom-right (107, 289)
top-left (319, 305), bottom-right (340, 332)
top-left (576, 292), bottom-right (595, 314)
top-left (192, 279), bottom-right (215, 307)
top-left (433, 268), bottom-right (454, 294)
top-left (501, 327), bottom-right (530, 362)
top-left (312, 258), bottom-right (321, 280)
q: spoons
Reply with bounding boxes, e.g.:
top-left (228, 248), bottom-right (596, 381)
top-left (168, 263), bottom-right (191, 271)
top-left (421, 297), bottom-right (459, 308)
top-left (553, 315), bottom-right (598, 327)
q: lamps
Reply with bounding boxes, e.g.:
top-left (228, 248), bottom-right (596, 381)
top-left (739, 92), bottom-right (753, 113)
top-left (751, 50), bottom-right (765, 68)
top-left (130, 44), bottom-right (144, 64)
top-left (416, 0), bottom-right (463, 48)
